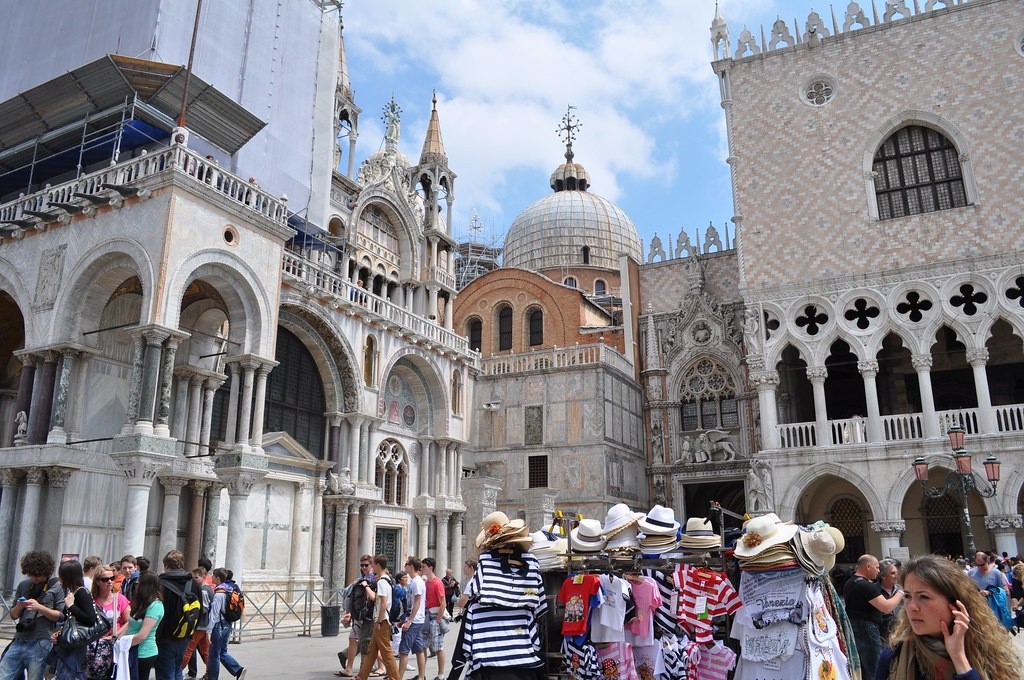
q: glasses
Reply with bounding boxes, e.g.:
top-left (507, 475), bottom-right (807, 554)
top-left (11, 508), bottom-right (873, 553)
top-left (360, 564), bottom-right (370, 567)
top-left (976, 562), bottom-right (986, 566)
top-left (28, 573), bottom-right (43, 577)
top-left (95, 576), bottom-right (115, 582)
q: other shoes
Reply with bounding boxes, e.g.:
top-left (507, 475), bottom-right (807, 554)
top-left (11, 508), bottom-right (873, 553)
top-left (236, 667), bottom-right (247, 680)
top-left (181, 673), bottom-right (196, 680)
top-left (338, 652), bottom-right (347, 668)
top-left (199, 674), bottom-right (208, 680)
top-left (351, 676), bottom-right (358, 680)
top-left (384, 651), bottom-right (445, 680)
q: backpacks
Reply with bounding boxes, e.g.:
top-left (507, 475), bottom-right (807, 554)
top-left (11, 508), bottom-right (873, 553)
top-left (160, 578), bottom-right (200, 640)
top-left (214, 589), bottom-right (243, 622)
top-left (378, 571), bottom-right (407, 622)
top-left (348, 579), bottom-right (374, 620)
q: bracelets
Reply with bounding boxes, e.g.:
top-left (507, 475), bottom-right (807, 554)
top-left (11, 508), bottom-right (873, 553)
top-left (898, 590), bottom-right (904, 596)
top-left (406, 620), bottom-right (411, 625)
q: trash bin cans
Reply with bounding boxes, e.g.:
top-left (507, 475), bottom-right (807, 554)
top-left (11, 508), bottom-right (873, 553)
top-left (320, 605), bottom-right (341, 636)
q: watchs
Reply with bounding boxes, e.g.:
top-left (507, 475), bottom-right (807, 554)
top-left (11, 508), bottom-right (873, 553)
top-left (377, 621), bottom-right (381, 625)
top-left (365, 585), bottom-right (370, 588)
top-left (111, 635), bottom-right (117, 641)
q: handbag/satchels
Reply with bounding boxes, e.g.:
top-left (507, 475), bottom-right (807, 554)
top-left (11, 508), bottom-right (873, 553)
top-left (59, 587), bottom-right (112, 649)
top-left (451, 591), bottom-right (457, 603)
top-left (82, 636), bottom-right (112, 678)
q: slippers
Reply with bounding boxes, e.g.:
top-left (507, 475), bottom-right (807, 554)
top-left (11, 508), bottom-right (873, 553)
top-left (369, 669), bottom-right (387, 677)
top-left (333, 671), bottom-right (353, 677)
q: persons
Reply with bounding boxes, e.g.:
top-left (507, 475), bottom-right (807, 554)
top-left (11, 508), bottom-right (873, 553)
top-left (872, 556), bottom-right (1023, 680)
top-left (332, 554), bottom-right (460, 680)
top-left (695, 322), bottom-right (710, 342)
top-left (748, 468), bottom-right (774, 511)
top-left (831, 554), bottom-right (905, 680)
top-left (464, 557), bottom-right (478, 580)
top-left (241, 178), bottom-right (260, 206)
top-left (198, 155), bottom-right (214, 183)
top-left (950, 551), bottom-right (1024, 637)
top-left (0, 550), bottom-right (247, 680)
top-left (652, 430), bottom-right (662, 464)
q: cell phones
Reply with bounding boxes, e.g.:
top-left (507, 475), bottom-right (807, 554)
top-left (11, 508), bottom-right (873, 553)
top-left (19, 598), bottom-right (26, 601)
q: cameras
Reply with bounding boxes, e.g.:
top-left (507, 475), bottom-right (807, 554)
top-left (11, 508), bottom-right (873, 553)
top-left (15, 617), bottom-right (35, 632)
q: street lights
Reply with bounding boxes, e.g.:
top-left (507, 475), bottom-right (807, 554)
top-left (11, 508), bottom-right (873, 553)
top-left (911, 422), bottom-right (1001, 566)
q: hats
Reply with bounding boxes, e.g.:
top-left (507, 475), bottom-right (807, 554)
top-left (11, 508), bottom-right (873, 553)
top-left (474, 500), bottom-right (845, 578)
